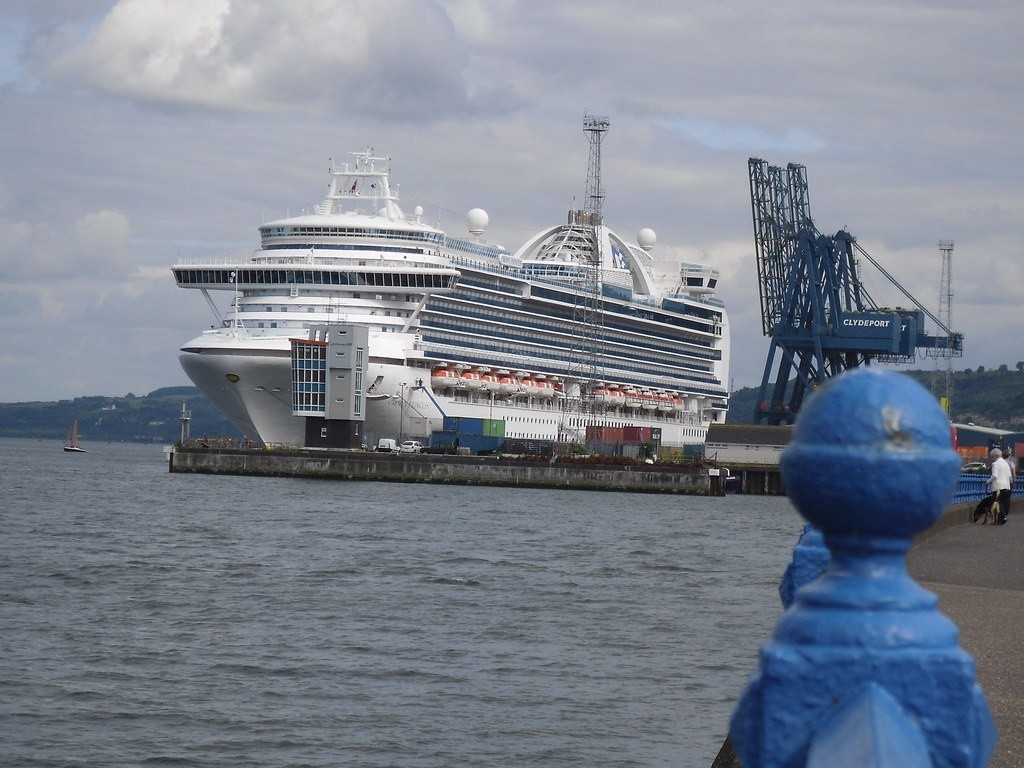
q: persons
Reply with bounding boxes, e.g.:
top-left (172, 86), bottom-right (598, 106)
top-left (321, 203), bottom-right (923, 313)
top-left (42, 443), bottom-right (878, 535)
top-left (1001, 450), bottom-right (1015, 514)
top-left (986, 448), bottom-right (1013, 526)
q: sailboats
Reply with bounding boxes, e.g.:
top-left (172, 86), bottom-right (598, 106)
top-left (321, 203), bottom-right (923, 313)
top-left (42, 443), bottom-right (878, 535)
top-left (64, 419), bottom-right (87, 452)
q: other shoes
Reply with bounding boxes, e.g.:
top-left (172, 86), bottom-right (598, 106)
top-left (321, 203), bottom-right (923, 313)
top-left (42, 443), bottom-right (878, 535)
top-left (1001, 516), bottom-right (1008, 524)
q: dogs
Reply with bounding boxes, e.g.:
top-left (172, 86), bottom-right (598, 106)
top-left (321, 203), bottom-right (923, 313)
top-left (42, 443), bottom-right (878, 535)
top-left (974, 489), bottom-right (1000, 526)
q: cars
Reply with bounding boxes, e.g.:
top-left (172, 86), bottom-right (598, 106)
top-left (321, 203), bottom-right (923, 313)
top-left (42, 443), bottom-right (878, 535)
top-left (378, 438), bottom-right (424, 453)
top-left (961, 461), bottom-right (986, 471)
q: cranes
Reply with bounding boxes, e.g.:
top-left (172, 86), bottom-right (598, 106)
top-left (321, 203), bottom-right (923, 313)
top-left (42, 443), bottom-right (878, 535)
top-left (747, 156), bottom-right (964, 426)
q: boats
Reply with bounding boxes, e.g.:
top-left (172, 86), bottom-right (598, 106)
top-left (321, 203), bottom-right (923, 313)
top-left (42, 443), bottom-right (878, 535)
top-left (431, 362), bottom-right (688, 412)
top-left (170, 145), bottom-right (730, 460)
